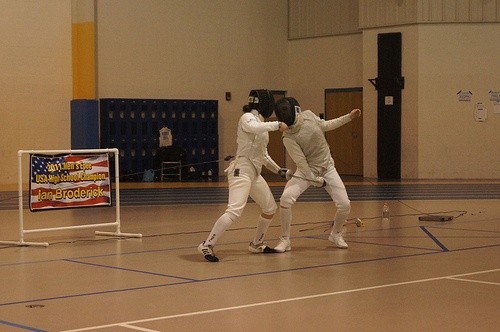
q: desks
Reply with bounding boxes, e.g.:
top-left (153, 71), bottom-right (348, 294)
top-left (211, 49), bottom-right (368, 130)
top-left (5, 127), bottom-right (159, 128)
top-left (161, 161), bottom-right (182, 181)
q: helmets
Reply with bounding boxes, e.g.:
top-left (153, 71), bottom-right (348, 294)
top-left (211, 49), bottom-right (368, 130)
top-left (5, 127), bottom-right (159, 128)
top-left (274, 97), bottom-right (300, 126)
top-left (249, 88), bottom-right (274, 117)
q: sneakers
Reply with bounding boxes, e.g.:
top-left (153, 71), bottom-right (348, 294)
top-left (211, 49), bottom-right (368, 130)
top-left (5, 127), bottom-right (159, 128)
top-left (328, 231), bottom-right (349, 249)
top-left (274, 238), bottom-right (292, 253)
top-left (248, 242), bottom-right (276, 253)
top-left (197, 241), bottom-right (219, 262)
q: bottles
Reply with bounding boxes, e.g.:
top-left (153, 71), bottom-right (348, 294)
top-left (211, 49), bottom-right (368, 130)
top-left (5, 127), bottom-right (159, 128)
top-left (382, 201), bottom-right (389, 220)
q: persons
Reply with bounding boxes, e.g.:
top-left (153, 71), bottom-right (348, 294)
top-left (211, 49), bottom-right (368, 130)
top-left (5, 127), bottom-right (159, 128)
top-left (196, 88), bottom-right (287, 263)
top-left (272, 97), bottom-right (361, 254)
